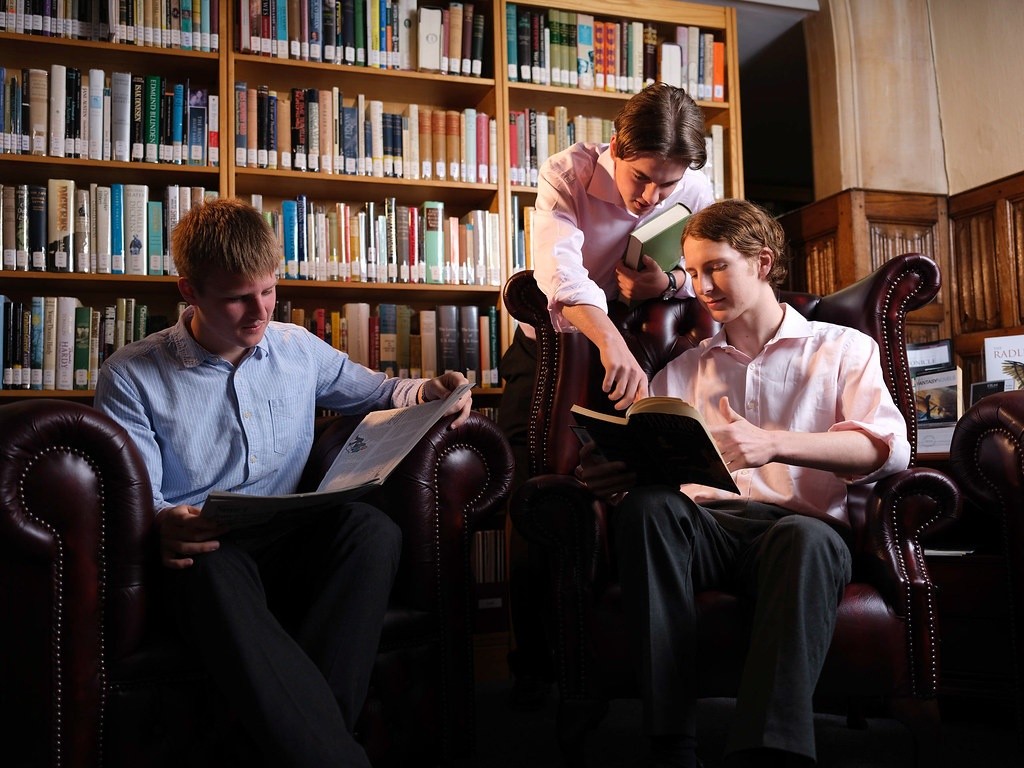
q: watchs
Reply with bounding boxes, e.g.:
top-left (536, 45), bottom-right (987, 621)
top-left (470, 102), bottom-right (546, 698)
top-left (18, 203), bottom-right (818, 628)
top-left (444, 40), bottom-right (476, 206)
top-left (654, 272), bottom-right (678, 305)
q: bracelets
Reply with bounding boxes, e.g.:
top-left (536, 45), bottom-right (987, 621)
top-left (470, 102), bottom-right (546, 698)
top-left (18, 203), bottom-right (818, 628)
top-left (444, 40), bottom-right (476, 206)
top-left (421, 379), bottom-right (433, 403)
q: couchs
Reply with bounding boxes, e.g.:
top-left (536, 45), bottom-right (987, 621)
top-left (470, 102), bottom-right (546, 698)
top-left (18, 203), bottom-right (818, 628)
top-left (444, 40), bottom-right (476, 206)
top-left (950, 391), bottom-right (1024, 768)
top-left (503, 253), bottom-right (960, 768)
top-left (0, 397), bottom-right (514, 768)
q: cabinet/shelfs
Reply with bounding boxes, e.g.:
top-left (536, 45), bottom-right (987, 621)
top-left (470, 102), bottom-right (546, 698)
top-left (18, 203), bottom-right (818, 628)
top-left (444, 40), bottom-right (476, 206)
top-left (0, 0), bottom-right (745, 403)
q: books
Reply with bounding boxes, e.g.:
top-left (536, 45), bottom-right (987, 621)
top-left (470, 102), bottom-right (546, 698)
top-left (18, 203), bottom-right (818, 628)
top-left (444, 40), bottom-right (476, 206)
top-left (904, 331), bottom-right (1024, 454)
top-left (570, 395), bottom-right (742, 496)
top-left (2, 1), bottom-right (732, 196)
top-left (617, 202), bottom-right (692, 312)
top-left (200, 379), bottom-right (477, 534)
top-left (0, 197), bottom-right (539, 393)
top-left (470, 406), bottom-right (499, 424)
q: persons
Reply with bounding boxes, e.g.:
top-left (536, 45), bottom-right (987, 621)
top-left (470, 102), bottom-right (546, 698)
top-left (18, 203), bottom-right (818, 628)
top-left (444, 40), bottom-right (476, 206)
top-left (494, 83), bottom-right (722, 719)
top-left (89, 197), bottom-right (472, 768)
top-left (571, 198), bottom-right (911, 768)
